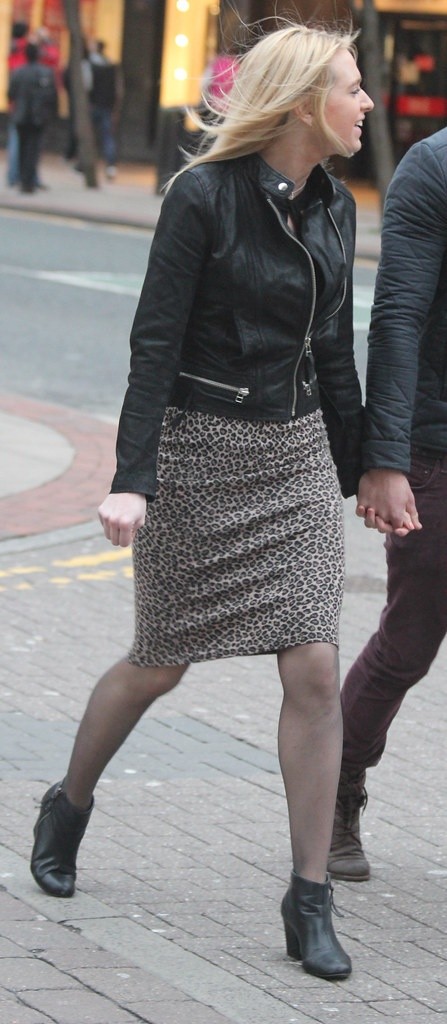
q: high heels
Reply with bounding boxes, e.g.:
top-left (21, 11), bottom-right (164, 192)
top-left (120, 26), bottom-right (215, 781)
top-left (30, 778), bottom-right (95, 898)
top-left (280, 870), bottom-right (352, 982)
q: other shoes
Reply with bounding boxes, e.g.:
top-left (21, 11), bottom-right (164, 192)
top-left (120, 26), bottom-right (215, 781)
top-left (104, 164), bottom-right (119, 186)
top-left (7, 174), bottom-right (45, 195)
top-left (73, 160), bottom-right (83, 173)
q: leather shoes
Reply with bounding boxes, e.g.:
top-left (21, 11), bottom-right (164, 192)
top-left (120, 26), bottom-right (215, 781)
top-left (325, 781), bottom-right (370, 883)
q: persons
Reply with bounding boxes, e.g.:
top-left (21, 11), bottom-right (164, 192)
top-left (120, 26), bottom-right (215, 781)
top-left (7, 21), bottom-right (114, 194)
top-left (201, 51), bottom-right (238, 101)
top-left (31, 21), bottom-right (447, 982)
